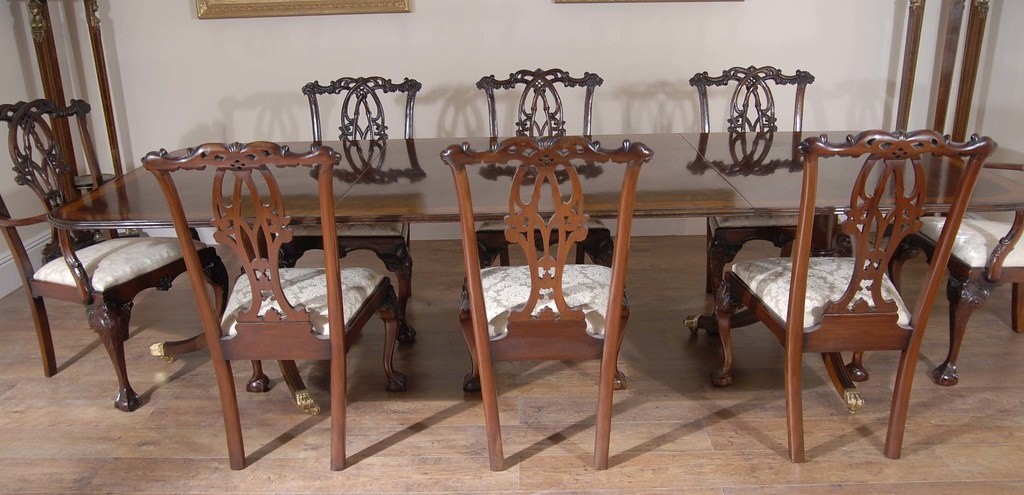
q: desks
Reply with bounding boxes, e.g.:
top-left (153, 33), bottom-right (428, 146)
top-left (44, 129), bottom-right (1012, 419)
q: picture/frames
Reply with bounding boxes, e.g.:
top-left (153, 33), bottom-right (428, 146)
top-left (192, 0), bottom-right (413, 20)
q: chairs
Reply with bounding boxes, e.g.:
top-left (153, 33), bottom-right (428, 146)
top-left (476, 67), bottom-right (614, 269)
top-left (718, 137), bottom-right (994, 462)
top-left (443, 138), bottom-right (655, 473)
top-left (889, 143), bottom-right (1024, 387)
top-left (0, 98), bottom-right (229, 412)
top-left (688, 65), bottom-right (839, 296)
top-left (277, 76), bottom-right (421, 345)
top-left (139, 139), bottom-right (416, 475)
